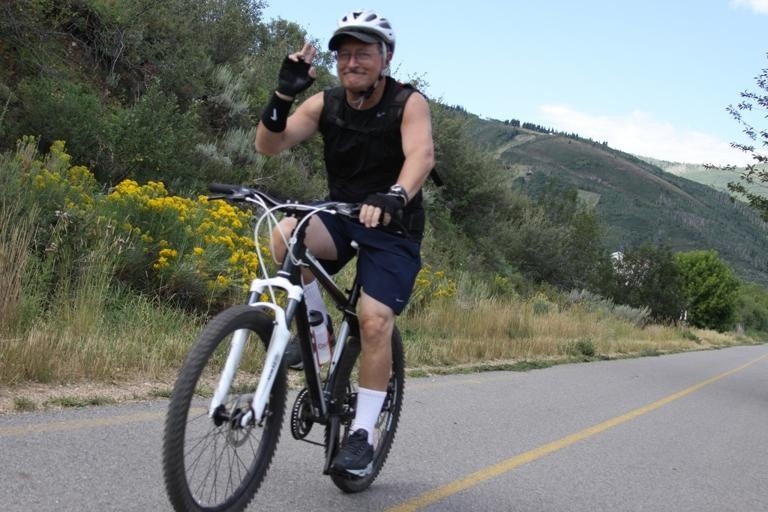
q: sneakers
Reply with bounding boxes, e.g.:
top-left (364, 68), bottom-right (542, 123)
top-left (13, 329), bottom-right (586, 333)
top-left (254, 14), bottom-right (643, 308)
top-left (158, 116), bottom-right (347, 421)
top-left (286, 314), bottom-right (335, 370)
top-left (331, 427), bottom-right (375, 478)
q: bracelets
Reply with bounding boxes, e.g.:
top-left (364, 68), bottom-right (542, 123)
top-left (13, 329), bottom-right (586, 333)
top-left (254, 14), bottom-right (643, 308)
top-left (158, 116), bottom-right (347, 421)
top-left (261, 92), bottom-right (294, 133)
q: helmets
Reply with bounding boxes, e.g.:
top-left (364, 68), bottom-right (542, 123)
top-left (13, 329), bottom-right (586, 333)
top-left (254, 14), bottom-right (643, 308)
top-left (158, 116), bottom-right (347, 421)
top-left (331, 9), bottom-right (395, 44)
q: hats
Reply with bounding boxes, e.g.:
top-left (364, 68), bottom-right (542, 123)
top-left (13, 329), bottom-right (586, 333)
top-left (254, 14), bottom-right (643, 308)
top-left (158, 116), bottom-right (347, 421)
top-left (327, 31), bottom-right (381, 51)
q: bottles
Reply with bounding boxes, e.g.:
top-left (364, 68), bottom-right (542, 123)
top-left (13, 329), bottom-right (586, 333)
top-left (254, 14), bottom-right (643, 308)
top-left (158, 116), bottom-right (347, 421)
top-left (308, 310), bottom-right (334, 367)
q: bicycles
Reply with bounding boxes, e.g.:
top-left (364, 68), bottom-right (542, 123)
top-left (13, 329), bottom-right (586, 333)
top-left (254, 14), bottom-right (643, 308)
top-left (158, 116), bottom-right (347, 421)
top-left (159, 181), bottom-right (408, 512)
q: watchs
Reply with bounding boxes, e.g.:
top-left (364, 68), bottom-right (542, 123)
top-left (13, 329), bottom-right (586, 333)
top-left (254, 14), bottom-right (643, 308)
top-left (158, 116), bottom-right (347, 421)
top-left (388, 184), bottom-right (409, 208)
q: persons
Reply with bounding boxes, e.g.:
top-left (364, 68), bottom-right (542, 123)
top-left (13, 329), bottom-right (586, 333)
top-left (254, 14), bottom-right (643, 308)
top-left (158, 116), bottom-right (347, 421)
top-left (252, 9), bottom-right (438, 470)
